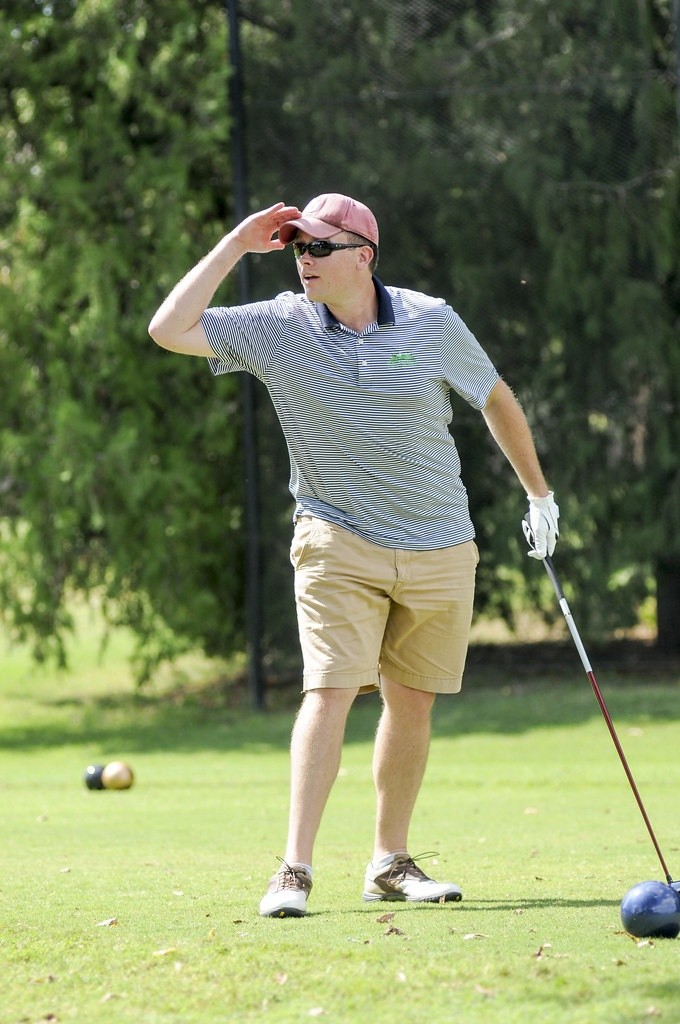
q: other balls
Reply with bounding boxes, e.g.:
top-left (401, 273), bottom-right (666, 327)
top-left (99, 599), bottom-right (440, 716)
top-left (101, 761), bottom-right (134, 790)
top-left (84, 763), bottom-right (106, 790)
top-left (620, 880), bottom-right (680, 939)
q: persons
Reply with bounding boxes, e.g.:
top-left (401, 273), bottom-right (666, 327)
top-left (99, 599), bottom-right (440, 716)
top-left (146, 193), bottom-right (558, 918)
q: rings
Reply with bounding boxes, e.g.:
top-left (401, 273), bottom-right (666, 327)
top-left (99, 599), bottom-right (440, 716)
top-left (277, 222), bottom-right (279, 227)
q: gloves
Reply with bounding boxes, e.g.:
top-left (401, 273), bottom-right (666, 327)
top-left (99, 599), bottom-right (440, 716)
top-left (521, 491), bottom-right (560, 560)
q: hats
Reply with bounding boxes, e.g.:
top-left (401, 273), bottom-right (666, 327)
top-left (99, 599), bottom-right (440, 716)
top-left (278, 193), bottom-right (378, 247)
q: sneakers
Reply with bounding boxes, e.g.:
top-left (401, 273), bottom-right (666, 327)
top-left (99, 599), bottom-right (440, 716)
top-left (362, 852), bottom-right (463, 903)
top-left (259, 856), bottom-right (313, 917)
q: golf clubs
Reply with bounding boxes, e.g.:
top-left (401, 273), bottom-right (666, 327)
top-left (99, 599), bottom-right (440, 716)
top-left (523, 510), bottom-right (680, 899)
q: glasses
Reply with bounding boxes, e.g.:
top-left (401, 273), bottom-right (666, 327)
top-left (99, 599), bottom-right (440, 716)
top-left (292, 241), bottom-right (376, 259)
